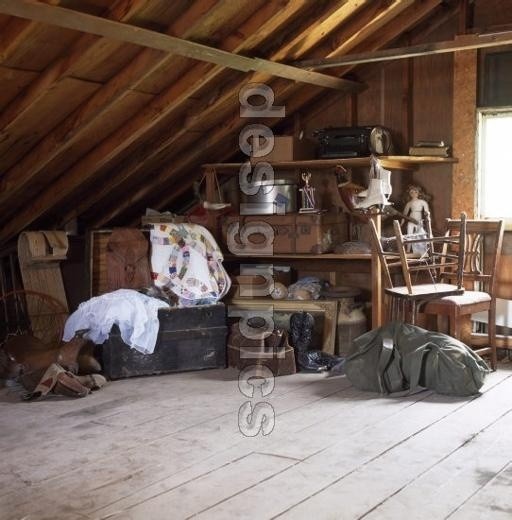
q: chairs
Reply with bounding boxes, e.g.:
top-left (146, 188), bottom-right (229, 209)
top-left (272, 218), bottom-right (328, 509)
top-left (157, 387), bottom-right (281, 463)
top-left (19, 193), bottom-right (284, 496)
top-left (369, 212), bottom-right (469, 322)
top-left (420, 219), bottom-right (506, 370)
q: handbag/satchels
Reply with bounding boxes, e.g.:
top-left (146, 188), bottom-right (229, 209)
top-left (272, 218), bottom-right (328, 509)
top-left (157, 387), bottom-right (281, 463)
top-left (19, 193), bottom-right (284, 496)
top-left (228, 322), bottom-right (297, 377)
top-left (344, 323), bottom-right (493, 397)
top-left (307, 126), bottom-right (394, 159)
top-left (106, 227), bottom-right (150, 292)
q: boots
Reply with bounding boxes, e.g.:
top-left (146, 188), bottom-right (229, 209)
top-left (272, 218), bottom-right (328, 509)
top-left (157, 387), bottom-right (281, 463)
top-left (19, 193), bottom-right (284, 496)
top-left (354, 180), bottom-right (385, 212)
top-left (357, 170), bottom-right (393, 199)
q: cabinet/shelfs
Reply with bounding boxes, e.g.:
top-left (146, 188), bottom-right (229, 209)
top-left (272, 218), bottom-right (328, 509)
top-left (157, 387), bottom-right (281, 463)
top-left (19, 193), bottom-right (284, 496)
top-left (200, 156), bottom-right (421, 332)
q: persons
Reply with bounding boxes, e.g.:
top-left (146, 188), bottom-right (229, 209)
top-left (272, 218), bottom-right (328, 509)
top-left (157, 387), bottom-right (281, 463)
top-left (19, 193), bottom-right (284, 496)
top-left (400, 184), bottom-right (433, 253)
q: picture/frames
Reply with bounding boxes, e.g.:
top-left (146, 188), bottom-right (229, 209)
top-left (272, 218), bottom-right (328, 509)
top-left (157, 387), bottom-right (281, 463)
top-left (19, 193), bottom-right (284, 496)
top-left (232, 298), bottom-right (336, 357)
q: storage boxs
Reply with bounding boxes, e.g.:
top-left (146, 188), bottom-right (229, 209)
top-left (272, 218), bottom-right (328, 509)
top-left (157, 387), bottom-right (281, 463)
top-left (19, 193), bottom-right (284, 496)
top-left (89, 230), bottom-right (230, 379)
top-left (249, 136), bottom-right (312, 163)
top-left (223, 214), bottom-right (349, 254)
